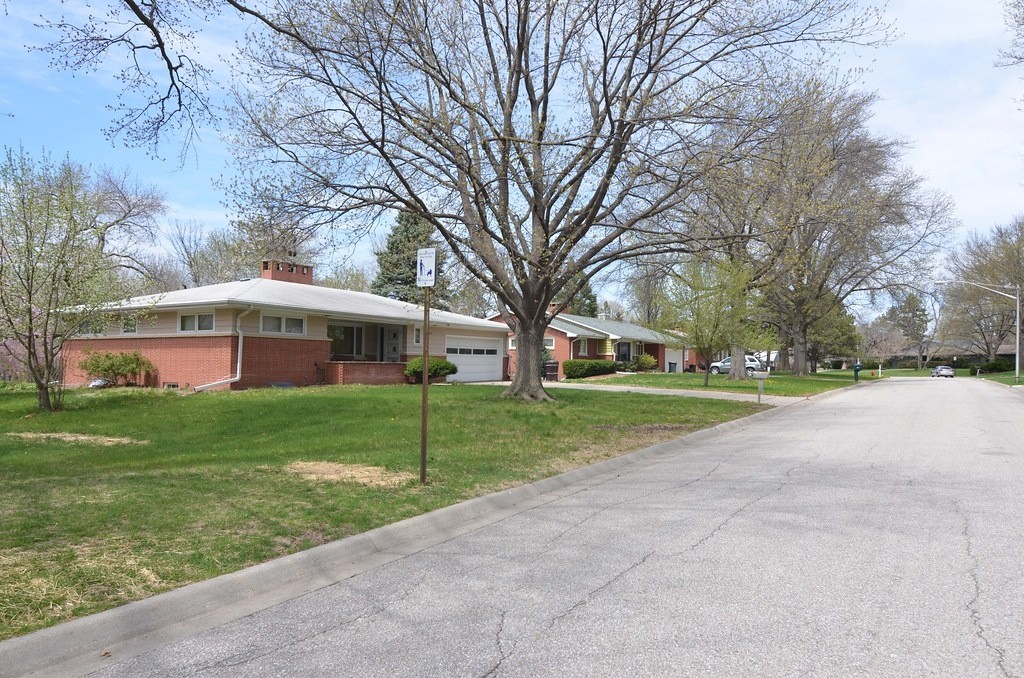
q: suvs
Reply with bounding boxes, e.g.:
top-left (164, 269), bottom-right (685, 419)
top-left (708, 355), bottom-right (762, 377)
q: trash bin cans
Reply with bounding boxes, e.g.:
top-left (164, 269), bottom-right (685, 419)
top-left (689, 364), bottom-right (696, 372)
top-left (668, 361), bottom-right (677, 373)
top-left (544, 360), bottom-right (558, 381)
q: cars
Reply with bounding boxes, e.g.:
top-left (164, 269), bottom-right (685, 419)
top-left (755, 350), bottom-right (795, 370)
top-left (931, 365), bottom-right (955, 377)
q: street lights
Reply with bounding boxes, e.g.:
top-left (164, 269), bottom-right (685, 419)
top-left (934, 278), bottom-right (1021, 384)
